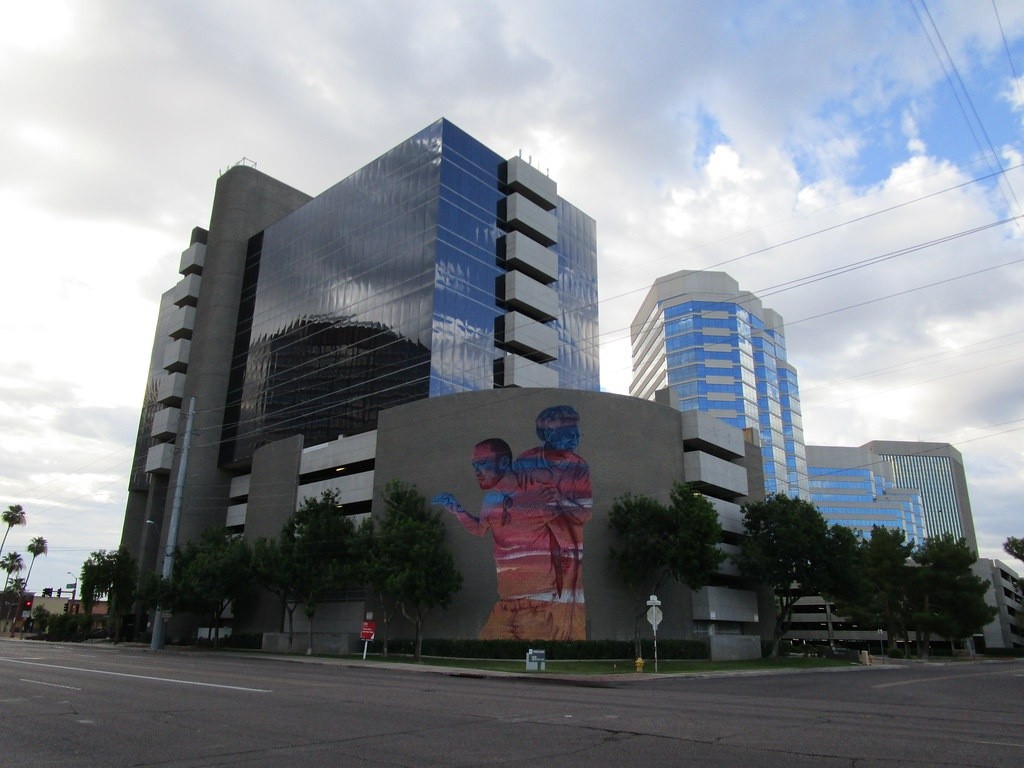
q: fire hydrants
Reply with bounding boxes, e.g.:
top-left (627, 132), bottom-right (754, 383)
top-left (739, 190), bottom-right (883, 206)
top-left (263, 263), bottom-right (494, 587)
top-left (636, 658), bottom-right (645, 673)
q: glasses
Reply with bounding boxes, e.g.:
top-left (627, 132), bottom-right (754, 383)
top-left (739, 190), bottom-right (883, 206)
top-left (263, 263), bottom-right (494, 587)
top-left (550, 428), bottom-right (581, 439)
top-left (500, 493), bottom-right (513, 527)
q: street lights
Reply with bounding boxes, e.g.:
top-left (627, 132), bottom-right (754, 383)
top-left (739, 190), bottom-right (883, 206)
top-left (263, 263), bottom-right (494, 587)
top-left (67, 571), bottom-right (78, 602)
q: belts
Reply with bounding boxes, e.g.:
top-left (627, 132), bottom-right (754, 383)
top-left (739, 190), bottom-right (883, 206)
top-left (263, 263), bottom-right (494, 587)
top-left (498, 598), bottom-right (553, 612)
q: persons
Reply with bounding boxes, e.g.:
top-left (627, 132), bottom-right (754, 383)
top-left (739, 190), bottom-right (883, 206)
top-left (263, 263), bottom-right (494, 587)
top-left (430, 405), bottom-right (593, 640)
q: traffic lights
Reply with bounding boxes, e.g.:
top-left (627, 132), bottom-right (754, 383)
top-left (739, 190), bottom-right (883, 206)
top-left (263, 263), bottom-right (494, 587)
top-left (64, 602), bottom-right (68, 613)
top-left (25, 594), bottom-right (34, 610)
top-left (49, 588), bottom-right (52, 597)
top-left (57, 588), bottom-right (61, 597)
top-left (42, 588), bottom-right (46, 597)
